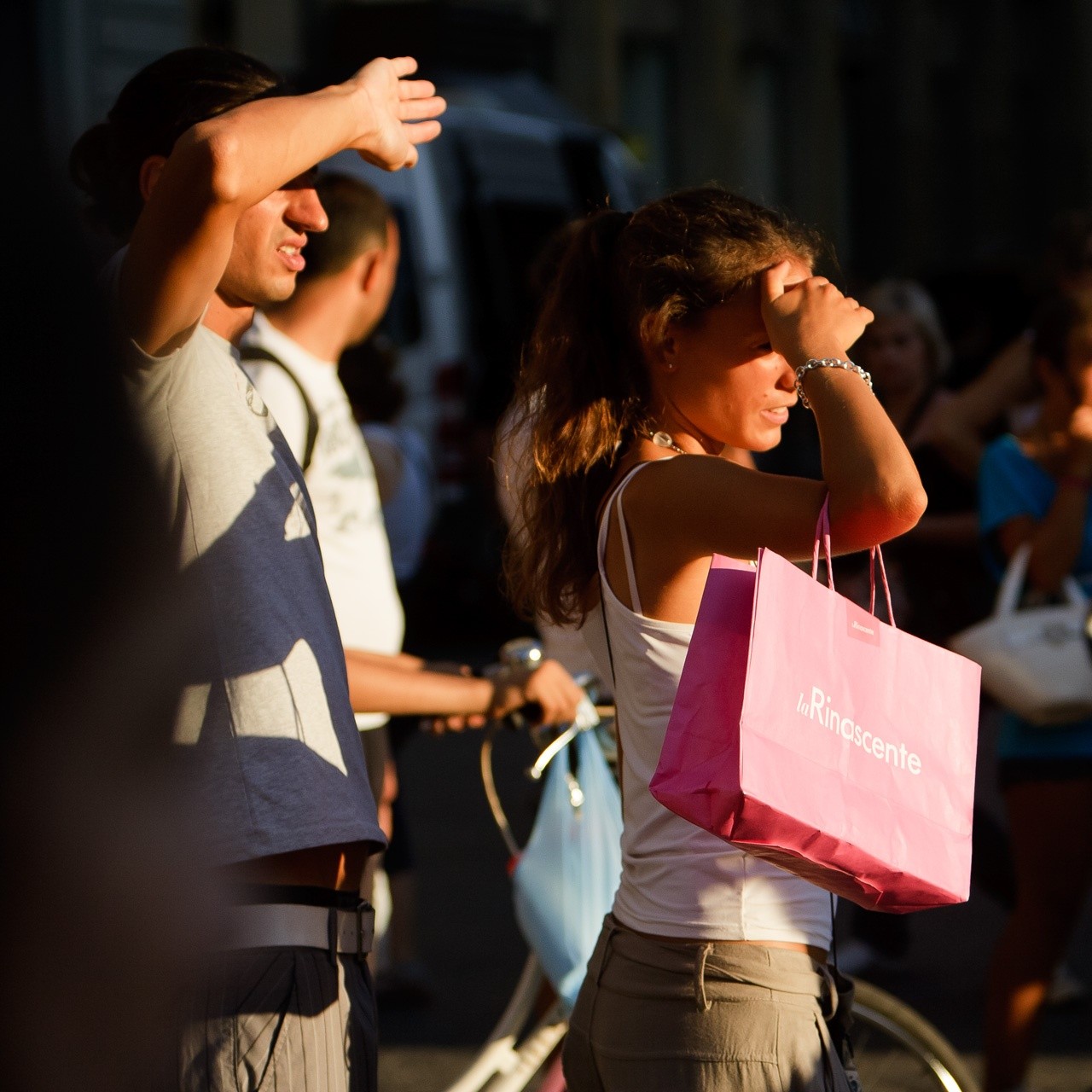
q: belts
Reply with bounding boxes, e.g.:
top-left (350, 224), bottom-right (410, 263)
top-left (222, 904), bottom-right (377, 954)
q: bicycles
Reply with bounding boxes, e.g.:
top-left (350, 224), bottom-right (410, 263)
top-left (373, 640), bottom-right (981, 1090)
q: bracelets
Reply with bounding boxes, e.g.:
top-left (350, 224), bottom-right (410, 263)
top-left (1058, 472), bottom-right (1089, 488)
top-left (795, 355), bottom-right (873, 411)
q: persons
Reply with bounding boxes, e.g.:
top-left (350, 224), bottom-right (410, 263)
top-left (231, 170), bottom-right (1092, 1092)
top-left (1, 48), bottom-right (450, 1092)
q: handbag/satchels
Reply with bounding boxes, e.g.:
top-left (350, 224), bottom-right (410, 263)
top-left (512, 673), bottom-right (624, 1008)
top-left (953, 540), bottom-right (1090, 727)
top-left (648, 483), bottom-right (981, 916)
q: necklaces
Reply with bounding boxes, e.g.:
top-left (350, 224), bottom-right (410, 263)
top-left (643, 426), bottom-right (687, 454)
